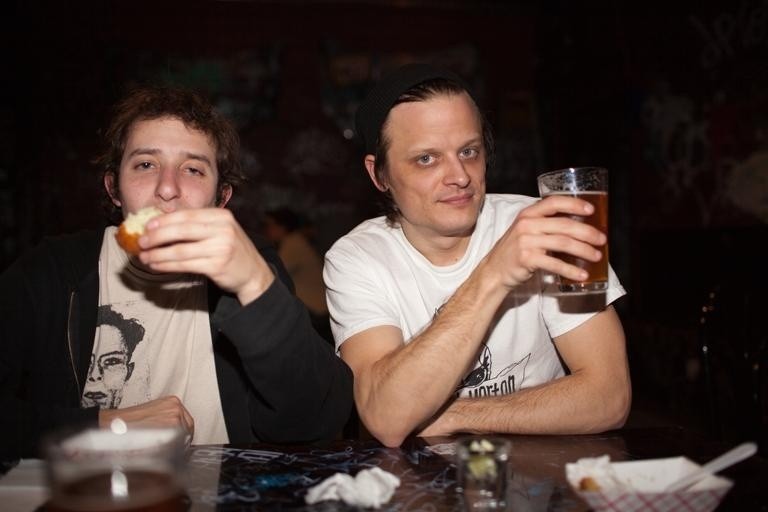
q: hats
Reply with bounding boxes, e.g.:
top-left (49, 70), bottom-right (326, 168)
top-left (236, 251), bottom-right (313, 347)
top-left (356, 64), bottom-right (463, 152)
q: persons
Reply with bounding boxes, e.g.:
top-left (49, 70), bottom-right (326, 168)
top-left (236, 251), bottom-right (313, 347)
top-left (81, 303), bottom-right (147, 410)
top-left (1, 76), bottom-right (355, 452)
top-left (320, 61), bottom-right (635, 452)
top-left (261, 203), bottom-right (336, 352)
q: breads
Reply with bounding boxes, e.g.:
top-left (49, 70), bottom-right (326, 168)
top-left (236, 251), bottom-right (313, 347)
top-left (113, 205), bottom-right (165, 256)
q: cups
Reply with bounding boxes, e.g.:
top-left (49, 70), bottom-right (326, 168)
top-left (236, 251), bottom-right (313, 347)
top-left (452, 432), bottom-right (513, 511)
top-left (535, 167), bottom-right (615, 298)
